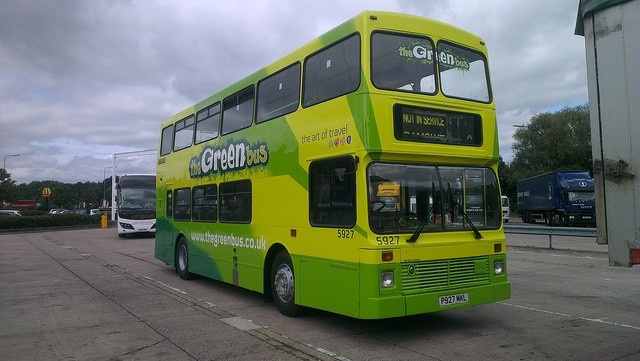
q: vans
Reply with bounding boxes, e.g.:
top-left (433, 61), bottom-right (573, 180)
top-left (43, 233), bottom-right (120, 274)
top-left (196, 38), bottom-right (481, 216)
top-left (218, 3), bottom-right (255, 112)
top-left (501, 196), bottom-right (510, 222)
top-left (49, 209), bottom-right (70, 214)
top-left (410, 196), bottom-right (433, 212)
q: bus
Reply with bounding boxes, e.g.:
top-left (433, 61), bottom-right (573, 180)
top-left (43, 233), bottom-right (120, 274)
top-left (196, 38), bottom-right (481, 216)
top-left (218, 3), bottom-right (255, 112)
top-left (156, 10), bottom-right (511, 319)
top-left (115, 173), bottom-right (156, 238)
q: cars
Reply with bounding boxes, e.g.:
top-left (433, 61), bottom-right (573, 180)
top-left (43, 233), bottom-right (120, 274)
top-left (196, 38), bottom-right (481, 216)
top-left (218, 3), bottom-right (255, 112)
top-left (0, 210), bottom-right (21, 216)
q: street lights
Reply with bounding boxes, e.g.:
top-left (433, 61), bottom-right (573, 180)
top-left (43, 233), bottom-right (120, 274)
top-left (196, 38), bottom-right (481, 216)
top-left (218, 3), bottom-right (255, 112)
top-left (104, 166), bottom-right (117, 199)
top-left (4, 154), bottom-right (21, 170)
top-left (513, 124), bottom-right (527, 127)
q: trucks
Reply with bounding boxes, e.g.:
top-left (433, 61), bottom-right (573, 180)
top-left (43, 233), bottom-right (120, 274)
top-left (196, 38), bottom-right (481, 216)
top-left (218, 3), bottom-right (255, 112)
top-left (376, 182), bottom-right (400, 211)
top-left (454, 178), bottom-right (483, 224)
top-left (517, 169), bottom-right (595, 226)
